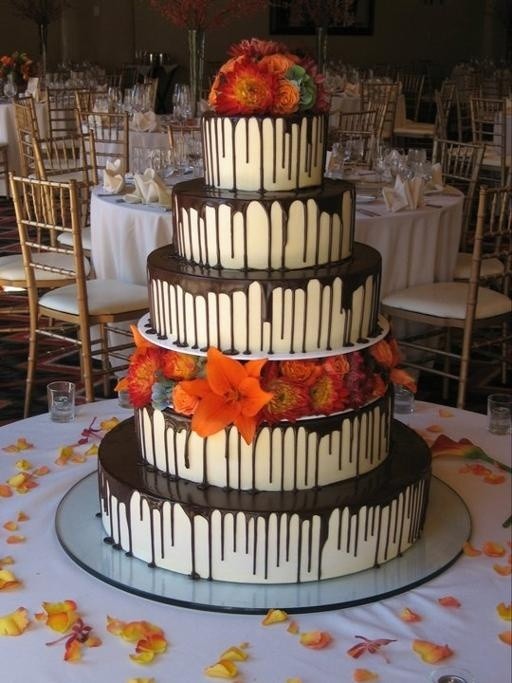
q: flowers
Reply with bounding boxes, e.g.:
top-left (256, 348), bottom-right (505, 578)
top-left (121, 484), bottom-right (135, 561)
top-left (154, 0), bottom-right (276, 30)
top-left (116, 326), bottom-right (421, 427)
top-left (0, 50), bottom-right (33, 81)
top-left (290, 0), bottom-right (357, 29)
top-left (208, 38), bottom-right (359, 116)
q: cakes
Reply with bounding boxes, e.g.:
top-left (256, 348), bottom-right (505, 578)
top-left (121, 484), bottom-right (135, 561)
top-left (96, 40), bottom-right (432, 585)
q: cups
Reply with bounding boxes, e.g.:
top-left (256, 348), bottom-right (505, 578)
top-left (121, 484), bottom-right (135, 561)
top-left (416, 160), bottom-right (431, 186)
top-left (171, 137), bottom-right (203, 179)
top-left (486, 392), bottom-right (512, 434)
top-left (117, 377), bottom-right (129, 406)
top-left (46, 380), bottom-right (75, 421)
top-left (394, 385), bottom-right (414, 414)
top-left (350, 140), bottom-right (363, 160)
top-left (331, 143), bottom-right (344, 165)
top-left (107, 81), bottom-right (153, 117)
top-left (398, 164), bottom-right (412, 180)
top-left (372, 157), bottom-right (386, 191)
top-left (132, 146), bottom-right (165, 171)
top-left (173, 82), bottom-right (193, 124)
top-left (388, 147), bottom-right (405, 171)
top-left (409, 149), bottom-right (427, 163)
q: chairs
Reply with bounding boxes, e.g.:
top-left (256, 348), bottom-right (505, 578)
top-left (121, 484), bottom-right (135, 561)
top-left (454, 170), bottom-right (512, 280)
top-left (8, 171), bottom-right (149, 419)
top-left (10, 94), bottom-right (94, 196)
top-left (32, 129), bottom-right (96, 254)
top-left (46, 86), bottom-right (95, 157)
top-left (393, 78), bottom-right (456, 152)
top-left (380, 184), bottom-right (512, 409)
top-left (471, 96), bottom-right (512, 187)
top-left (334, 106), bottom-right (379, 170)
top-left (359, 79), bottom-right (399, 152)
top-left (457, 76), bottom-right (480, 143)
top-left (167, 125), bottom-right (202, 155)
top-left (434, 90), bottom-right (447, 146)
top-left (397, 70), bottom-right (426, 120)
top-left (75, 108), bottom-right (127, 227)
top-left (0, 250), bottom-right (103, 383)
top-left (433, 135), bottom-right (485, 244)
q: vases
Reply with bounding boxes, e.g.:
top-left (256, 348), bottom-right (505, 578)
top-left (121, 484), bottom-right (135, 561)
top-left (4, 73), bottom-right (16, 98)
top-left (188, 30), bottom-right (205, 114)
top-left (317, 29), bottom-right (327, 74)
